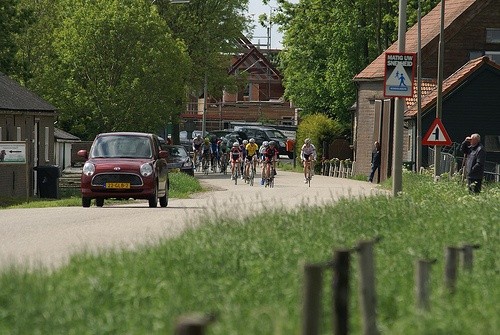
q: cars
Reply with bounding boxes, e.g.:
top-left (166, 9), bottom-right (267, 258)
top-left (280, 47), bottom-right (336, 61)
top-left (77, 131), bottom-right (169, 208)
top-left (167, 145), bottom-right (194, 177)
top-left (167, 130), bottom-right (216, 151)
top-left (221, 126), bottom-right (293, 159)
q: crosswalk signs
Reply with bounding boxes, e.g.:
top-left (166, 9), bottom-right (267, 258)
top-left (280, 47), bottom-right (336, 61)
top-left (384, 52), bottom-right (417, 98)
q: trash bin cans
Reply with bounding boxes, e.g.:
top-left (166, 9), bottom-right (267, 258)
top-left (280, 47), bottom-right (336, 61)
top-left (402, 161), bottom-right (415, 171)
top-left (33, 165), bottom-right (62, 199)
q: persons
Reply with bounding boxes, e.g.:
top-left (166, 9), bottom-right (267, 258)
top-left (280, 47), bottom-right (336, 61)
top-left (192, 134), bottom-right (258, 180)
top-left (167, 138), bottom-right (173, 145)
top-left (259, 141), bottom-right (279, 188)
top-left (301, 138), bottom-right (316, 183)
top-left (368, 141), bottom-right (381, 184)
top-left (460, 133), bottom-right (484, 194)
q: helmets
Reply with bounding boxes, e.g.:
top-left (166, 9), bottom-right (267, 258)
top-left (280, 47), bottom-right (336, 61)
top-left (269, 141), bottom-right (276, 146)
top-left (249, 138), bottom-right (255, 143)
top-left (263, 141), bottom-right (269, 146)
top-left (233, 142), bottom-right (239, 147)
top-left (197, 134), bottom-right (239, 142)
top-left (305, 138), bottom-right (311, 144)
top-left (243, 140), bottom-right (248, 145)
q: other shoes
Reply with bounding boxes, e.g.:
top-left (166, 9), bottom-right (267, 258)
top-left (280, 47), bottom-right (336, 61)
top-left (304, 178), bottom-right (308, 183)
top-left (241, 175), bottom-right (243, 180)
top-left (274, 170), bottom-right (277, 175)
top-left (231, 176), bottom-right (234, 180)
top-left (311, 170), bottom-right (314, 176)
top-left (261, 179), bottom-right (264, 185)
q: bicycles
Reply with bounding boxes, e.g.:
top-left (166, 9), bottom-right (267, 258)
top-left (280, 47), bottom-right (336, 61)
top-left (303, 159), bottom-right (314, 187)
top-left (195, 151), bottom-right (278, 189)
